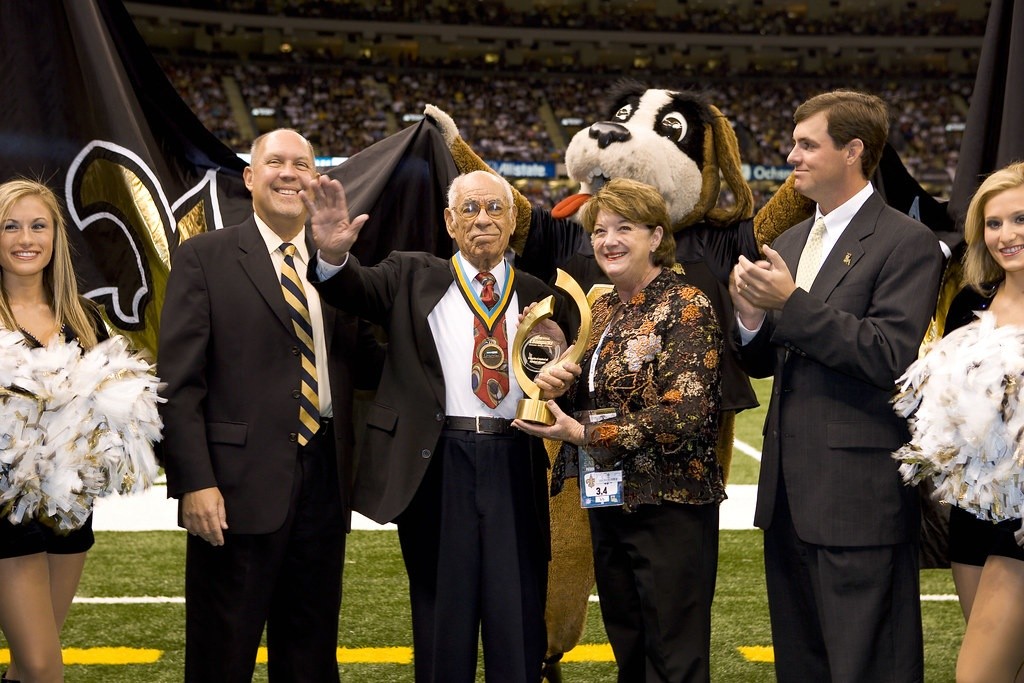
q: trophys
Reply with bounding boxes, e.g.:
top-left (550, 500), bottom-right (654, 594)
top-left (512, 267), bottom-right (592, 427)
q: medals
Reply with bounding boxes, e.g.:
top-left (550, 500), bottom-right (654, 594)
top-left (479, 342), bottom-right (505, 370)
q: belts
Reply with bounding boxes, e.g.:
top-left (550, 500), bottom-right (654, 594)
top-left (442, 416), bottom-right (527, 438)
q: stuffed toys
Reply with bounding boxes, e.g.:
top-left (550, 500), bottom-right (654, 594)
top-left (425, 80), bottom-right (817, 681)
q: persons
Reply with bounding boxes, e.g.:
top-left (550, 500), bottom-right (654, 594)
top-left (166, 52), bottom-right (976, 212)
top-left (512, 178), bottom-right (729, 682)
top-left (154, 129), bottom-right (355, 681)
top-left (0, 180), bottom-right (110, 683)
top-left (298, 168), bottom-right (583, 682)
top-left (729, 88), bottom-right (945, 683)
top-left (940, 160), bottom-right (1024, 683)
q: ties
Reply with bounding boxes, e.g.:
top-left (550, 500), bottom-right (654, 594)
top-left (795, 219), bottom-right (826, 293)
top-left (472, 274), bottom-right (510, 409)
top-left (278, 243), bottom-right (320, 447)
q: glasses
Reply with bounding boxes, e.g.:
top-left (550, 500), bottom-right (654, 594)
top-left (451, 200), bottom-right (511, 221)
